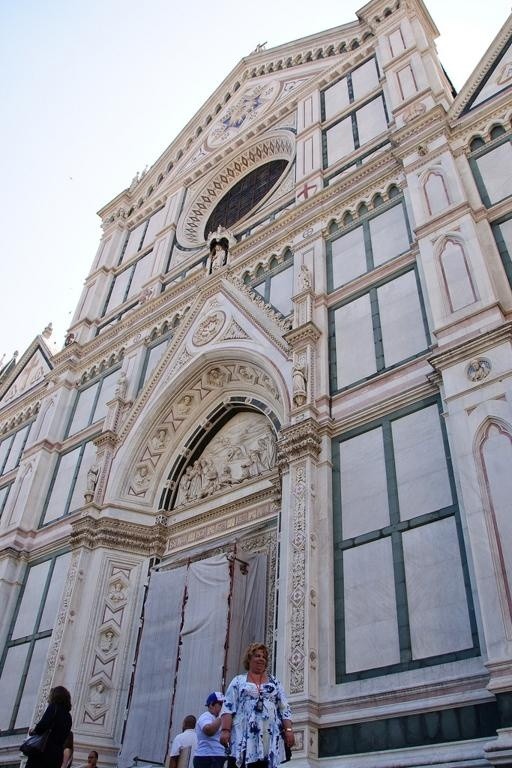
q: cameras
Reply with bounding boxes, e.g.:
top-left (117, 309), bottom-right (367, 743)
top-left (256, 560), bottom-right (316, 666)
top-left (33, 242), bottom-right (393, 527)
top-left (254, 696), bottom-right (264, 712)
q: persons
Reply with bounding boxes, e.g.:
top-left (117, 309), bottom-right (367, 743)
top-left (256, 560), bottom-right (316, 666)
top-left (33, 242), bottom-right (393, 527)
top-left (116, 371), bottom-right (128, 399)
top-left (169, 715), bottom-right (196, 768)
top-left (23, 686), bottom-right (100, 768)
top-left (192, 691), bottom-right (229, 768)
top-left (297, 263), bottom-right (312, 293)
top-left (219, 642), bottom-right (295, 768)
top-left (130, 367), bottom-right (278, 504)
top-left (212, 244), bottom-right (226, 274)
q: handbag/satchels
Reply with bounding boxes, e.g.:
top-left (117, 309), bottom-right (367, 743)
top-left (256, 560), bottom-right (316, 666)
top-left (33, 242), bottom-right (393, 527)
top-left (20, 734), bottom-right (44, 756)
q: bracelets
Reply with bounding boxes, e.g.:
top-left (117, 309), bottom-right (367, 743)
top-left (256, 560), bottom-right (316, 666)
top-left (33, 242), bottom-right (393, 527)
top-left (283, 728), bottom-right (293, 732)
top-left (220, 728), bottom-right (231, 733)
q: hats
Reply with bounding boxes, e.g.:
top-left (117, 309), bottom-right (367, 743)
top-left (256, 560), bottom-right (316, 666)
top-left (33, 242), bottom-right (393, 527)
top-left (206, 692), bottom-right (225, 705)
